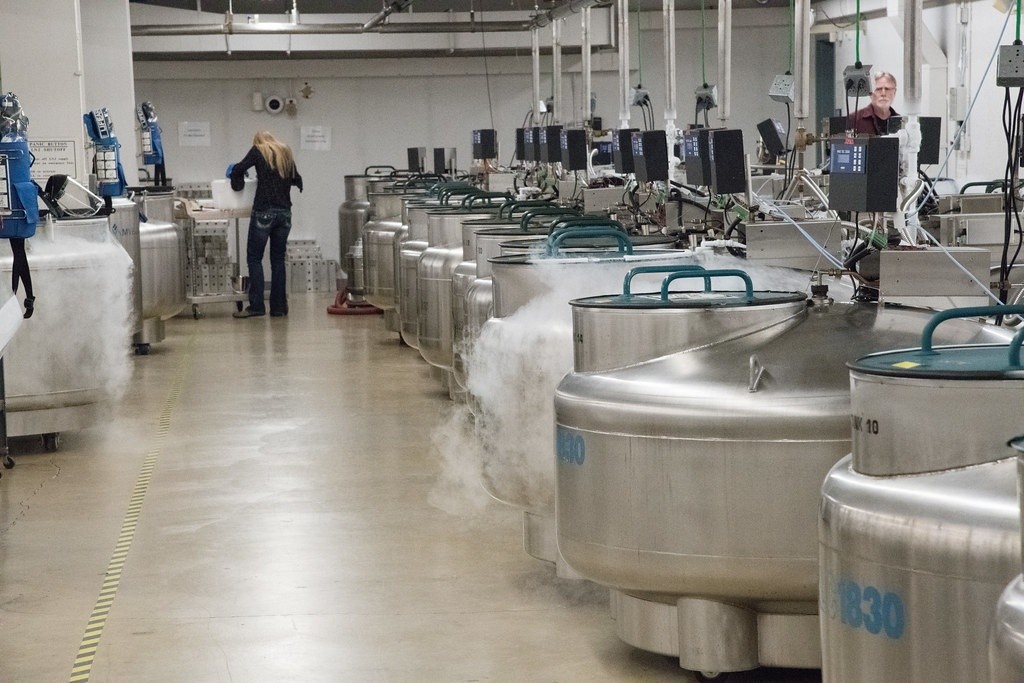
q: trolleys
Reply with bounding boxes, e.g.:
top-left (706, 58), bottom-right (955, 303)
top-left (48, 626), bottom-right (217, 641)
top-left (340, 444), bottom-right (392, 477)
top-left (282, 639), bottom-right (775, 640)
top-left (175, 197), bottom-right (249, 320)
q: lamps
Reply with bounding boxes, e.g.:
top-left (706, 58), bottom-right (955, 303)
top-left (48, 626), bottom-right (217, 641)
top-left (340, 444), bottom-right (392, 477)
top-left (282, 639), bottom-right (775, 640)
top-left (508, 101), bottom-right (548, 168)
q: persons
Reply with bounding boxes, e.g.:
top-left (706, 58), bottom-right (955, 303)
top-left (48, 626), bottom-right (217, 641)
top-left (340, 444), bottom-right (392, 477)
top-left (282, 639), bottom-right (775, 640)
top-left (845, 71), bottom-right (902, 136)
top-left (231, 129), bottom-right (303, 318)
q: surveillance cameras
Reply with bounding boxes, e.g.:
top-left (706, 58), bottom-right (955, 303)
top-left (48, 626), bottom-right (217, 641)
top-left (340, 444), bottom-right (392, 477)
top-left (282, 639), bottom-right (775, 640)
top-left (266, 96), bottom-right (284, 114)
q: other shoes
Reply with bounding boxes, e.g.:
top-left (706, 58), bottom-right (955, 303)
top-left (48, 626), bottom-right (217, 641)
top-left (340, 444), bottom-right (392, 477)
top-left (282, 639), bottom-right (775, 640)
top-left (232, 308), bottom-right (252, 318)
